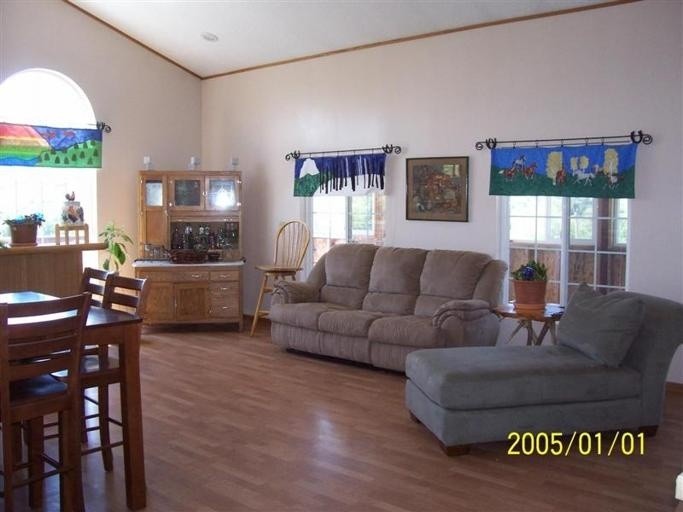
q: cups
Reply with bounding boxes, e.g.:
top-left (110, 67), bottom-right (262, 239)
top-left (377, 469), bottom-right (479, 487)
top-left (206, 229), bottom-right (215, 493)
top-left (143, 244), bottom-right (166, 259)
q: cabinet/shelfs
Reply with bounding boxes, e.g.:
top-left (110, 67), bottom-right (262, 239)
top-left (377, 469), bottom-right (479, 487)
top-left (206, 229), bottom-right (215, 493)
top-left (133, 169), bottom-right (247, 332)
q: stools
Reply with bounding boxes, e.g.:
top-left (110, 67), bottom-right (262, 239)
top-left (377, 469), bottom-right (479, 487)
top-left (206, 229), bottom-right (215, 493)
top-left (55, 223), bottom-right (88, 245)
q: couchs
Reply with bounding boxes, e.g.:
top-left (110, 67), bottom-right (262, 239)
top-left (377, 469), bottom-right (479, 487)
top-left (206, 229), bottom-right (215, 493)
top-left (269, 244), bottom-right (508, 372)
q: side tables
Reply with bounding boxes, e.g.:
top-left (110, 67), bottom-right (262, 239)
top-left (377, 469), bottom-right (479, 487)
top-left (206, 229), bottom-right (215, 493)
top-left (491, 303), bottom-right (565, 345)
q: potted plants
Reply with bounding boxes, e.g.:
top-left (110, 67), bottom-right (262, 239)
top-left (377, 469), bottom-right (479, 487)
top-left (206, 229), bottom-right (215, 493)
top-left (61, 191), bottom-right (84, 225)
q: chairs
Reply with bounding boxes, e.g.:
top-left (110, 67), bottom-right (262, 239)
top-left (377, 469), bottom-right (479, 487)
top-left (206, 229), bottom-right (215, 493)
top-left (0, 267), bottom-right (152, 512)
top-left (250, 220), bottom-right (309, 337)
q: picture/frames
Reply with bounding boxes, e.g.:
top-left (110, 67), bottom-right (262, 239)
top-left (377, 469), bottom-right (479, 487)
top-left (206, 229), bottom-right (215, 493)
top-left (405, 157), bottom-right (469, 222)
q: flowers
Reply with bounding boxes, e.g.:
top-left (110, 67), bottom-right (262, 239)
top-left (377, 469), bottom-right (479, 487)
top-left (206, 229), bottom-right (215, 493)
top-left (510, 255), bottom-right (550, 280)
top-left (3, 211), bottom-right (43, 229)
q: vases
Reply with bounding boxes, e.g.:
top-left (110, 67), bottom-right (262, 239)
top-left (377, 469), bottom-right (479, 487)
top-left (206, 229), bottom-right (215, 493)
top-left (10, 224), bottom-right (37, 243)
top-left (513, 280), bottom-right (546, 305)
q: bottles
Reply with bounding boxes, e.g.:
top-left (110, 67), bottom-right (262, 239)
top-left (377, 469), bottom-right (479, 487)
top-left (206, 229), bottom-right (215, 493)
top-left (171, 223), bottom-right (237, 249)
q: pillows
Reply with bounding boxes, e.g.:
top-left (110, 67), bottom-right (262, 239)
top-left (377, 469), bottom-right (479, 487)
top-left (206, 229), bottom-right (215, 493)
top-left (556, 281), bottom-right (646, 368)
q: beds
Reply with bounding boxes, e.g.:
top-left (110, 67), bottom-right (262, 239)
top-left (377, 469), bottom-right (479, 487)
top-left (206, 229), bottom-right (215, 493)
top-left (404, 291), bottom-right (683, 457)
